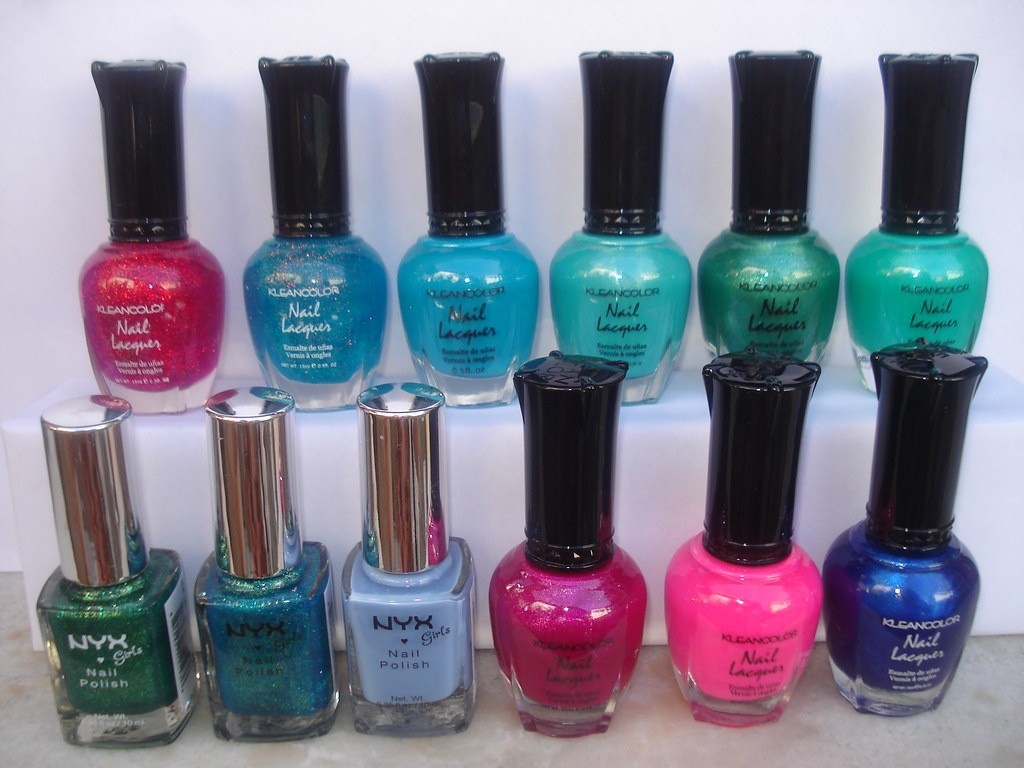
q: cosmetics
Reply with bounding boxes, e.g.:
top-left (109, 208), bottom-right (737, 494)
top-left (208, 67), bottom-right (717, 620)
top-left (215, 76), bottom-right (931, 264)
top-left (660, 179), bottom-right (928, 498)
top-left (844, 52), bottom-right (990, 394)
top-left (35, 394), bottom-right (201, 750)
top-left (548, 48), bottom-right (693, 405)
top-left (695, 49), bottom-right (842, 364)
top-left (488, 350), bottom-right (648, 738)
top-left (193, 385), bottom-right (340, 744)
top-left (663, 345), bottom-right (824, 729)
top-left (242, 55), bottom-right (388, 414)
top-left (76, 58), bottom-right (228, 418)
top-left (341, 381), bottom-right (478, 738)
top-left (395, 49), bottom-right (542, 409)
top-left (822, 336), bottom-right (989, 719)
